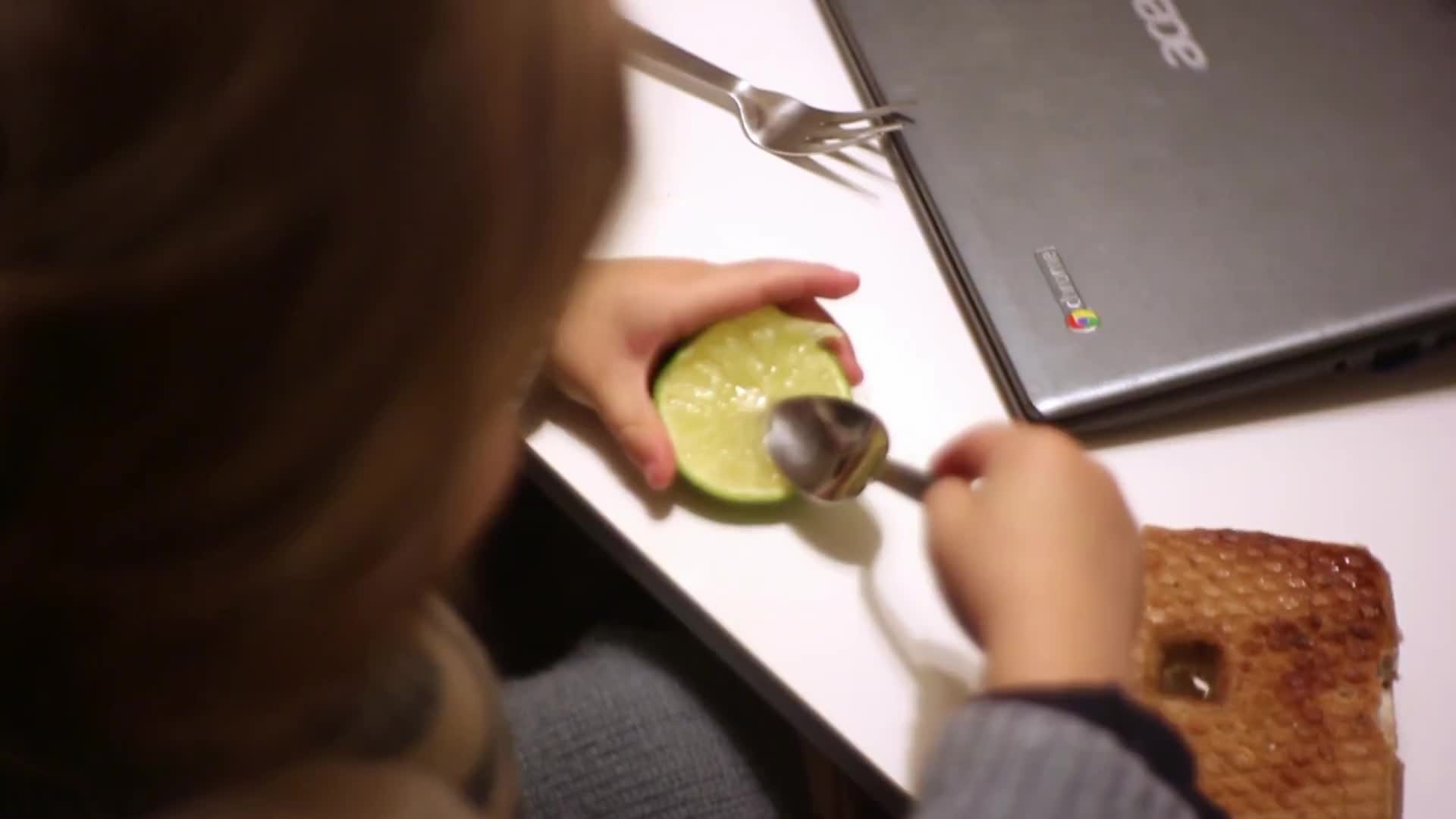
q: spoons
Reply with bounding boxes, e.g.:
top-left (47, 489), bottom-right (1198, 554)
top-left (764, 396), bottom-right (937, 512)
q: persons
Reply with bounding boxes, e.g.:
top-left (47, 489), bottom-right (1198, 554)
top-left (0, 0), bottom-right (1235, 816)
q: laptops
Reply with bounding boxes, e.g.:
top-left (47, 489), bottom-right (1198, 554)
top-left (812, 0), bottom-right (1455, 445)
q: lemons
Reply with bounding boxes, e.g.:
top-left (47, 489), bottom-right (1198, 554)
top-left (653, 302), bottom-right (854, 507)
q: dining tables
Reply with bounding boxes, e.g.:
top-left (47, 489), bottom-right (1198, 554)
top-left (514, 0), bottom-right (1456, 819)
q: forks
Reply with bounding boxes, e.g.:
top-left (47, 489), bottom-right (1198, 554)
top-left (638, 17), bottom-right (911, 157)
